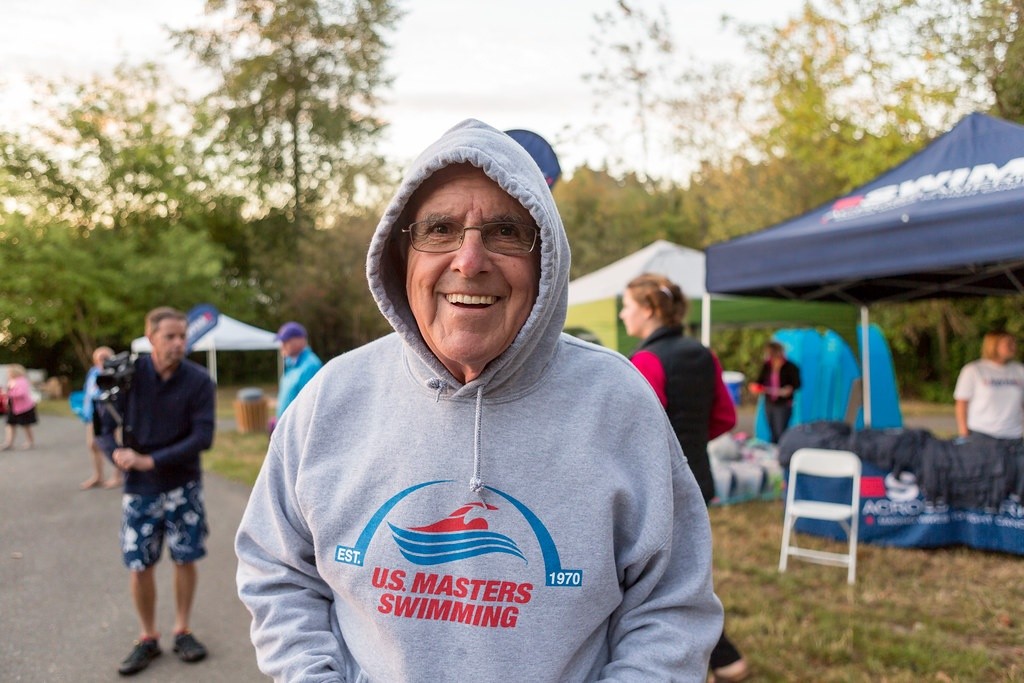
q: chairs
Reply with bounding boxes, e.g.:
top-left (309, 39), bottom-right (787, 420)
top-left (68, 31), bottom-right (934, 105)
top-left (777, 446), bottom-right (861, 585)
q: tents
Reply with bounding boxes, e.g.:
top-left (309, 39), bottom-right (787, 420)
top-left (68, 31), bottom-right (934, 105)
top-left (704, 109), bottom-right (1024, 428)
top-left (128, 305), bottom-right (281, 387)
top-left (567, 239), bottom-right (872, 428)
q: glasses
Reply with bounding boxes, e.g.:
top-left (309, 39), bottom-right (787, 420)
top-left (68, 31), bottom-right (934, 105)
top-left (400, 219), bottom-right (541, 256)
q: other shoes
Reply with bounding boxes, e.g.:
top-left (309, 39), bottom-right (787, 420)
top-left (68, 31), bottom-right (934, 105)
top-left (705, 665), bottom-right (752, 683)
top-left (118, 638), bottom-right (160, 674)
top-left (174, 632), bottom-right (207, 661)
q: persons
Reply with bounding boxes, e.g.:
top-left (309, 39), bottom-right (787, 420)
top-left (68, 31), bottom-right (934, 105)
top-left (0, 364), bottom-right (42, 451)
top-left (232, 116), bottom-right (727, 683)
top-left (617, 271), bottom-right (752, 683)
top-left (745, 337), bottom-right (803, 444)
top-left (952, 330), bottom-right (1024, 441)
top-left (269, 320), bottom-right (324, 438)
top-left (81, 304), bottom-right (217, 676)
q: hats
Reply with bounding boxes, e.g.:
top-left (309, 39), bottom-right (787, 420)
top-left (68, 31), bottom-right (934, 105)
top-left (272, 322), bottom-right (307, 342)
